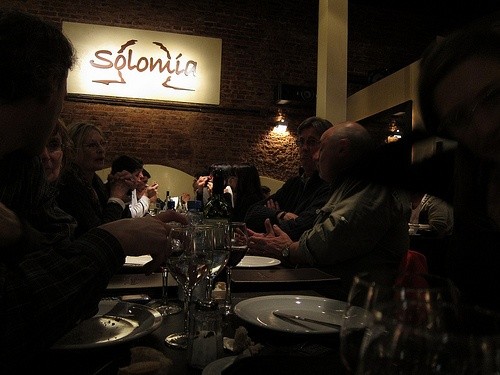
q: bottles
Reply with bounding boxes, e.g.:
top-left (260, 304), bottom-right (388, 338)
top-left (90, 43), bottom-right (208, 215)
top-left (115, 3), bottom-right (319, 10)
top-left (203, 169), bottom-right (232, 222)
top-left (146, 190), bottom-right (202, 217)
top-left (186, 297), bottom-right (223, 369)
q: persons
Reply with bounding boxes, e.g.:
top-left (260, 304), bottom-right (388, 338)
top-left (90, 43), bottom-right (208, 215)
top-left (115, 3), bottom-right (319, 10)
top-left (32, 19), bottom-right (500, 375)
top-left (0, 12), bottom-right (190, 375)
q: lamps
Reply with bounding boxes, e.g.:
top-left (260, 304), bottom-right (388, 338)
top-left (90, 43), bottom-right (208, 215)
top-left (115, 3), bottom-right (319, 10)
top-left (272, 105), bottom-right (288, 123)
top-left (384, 118), bottom-right (401, 143)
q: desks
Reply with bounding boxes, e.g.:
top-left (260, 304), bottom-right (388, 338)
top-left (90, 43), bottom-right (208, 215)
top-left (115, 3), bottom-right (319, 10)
top-left (105, 254), bottom-right (343, 298)
top-left (114, 290), bottom-right (322, 375)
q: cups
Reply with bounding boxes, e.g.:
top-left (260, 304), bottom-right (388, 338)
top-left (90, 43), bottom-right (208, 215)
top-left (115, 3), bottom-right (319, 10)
top-left (337, 272), bottom-right (496, 375)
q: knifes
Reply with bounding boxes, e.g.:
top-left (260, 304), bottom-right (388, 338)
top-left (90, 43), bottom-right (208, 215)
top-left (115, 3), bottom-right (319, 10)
top-left (272, 311), bottom-right (341, 329)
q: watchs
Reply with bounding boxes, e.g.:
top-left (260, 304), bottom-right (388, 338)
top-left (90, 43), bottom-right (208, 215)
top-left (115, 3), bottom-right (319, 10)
top-left (281, 242), bottom-right (294, 259)
top-left (277, 211), bottom-right (288, 224)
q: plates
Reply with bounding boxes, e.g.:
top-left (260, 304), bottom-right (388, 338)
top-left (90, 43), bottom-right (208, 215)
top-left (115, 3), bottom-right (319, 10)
top-left (235, 255), bottom-right (282, 268)
top-left (123, 255), bottom-right (153, 269)
top-left (51, 300), bottom-right (163, 350)
top-left (233, 295), bottom-right (372, 334)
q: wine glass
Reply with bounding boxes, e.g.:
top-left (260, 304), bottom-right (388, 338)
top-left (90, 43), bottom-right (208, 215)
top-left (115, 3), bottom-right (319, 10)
top-left (149, 217), bottom-right (247, 317)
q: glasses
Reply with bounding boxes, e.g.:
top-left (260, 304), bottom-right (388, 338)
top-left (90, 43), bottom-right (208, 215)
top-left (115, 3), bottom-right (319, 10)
top-left (296, 137), bottom-right (320, 148)
top-left (83, 141), bottom-right (108, 149)
